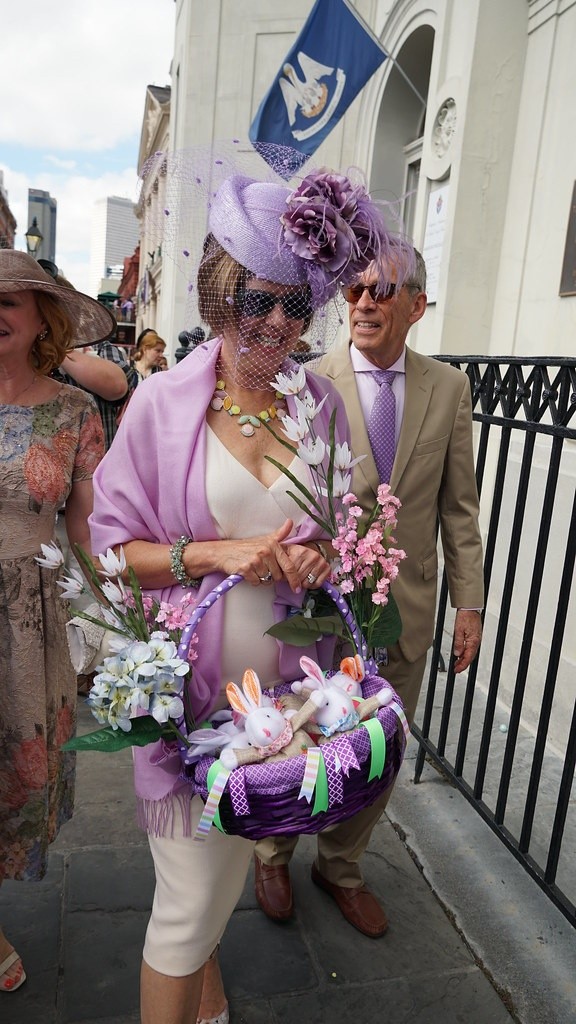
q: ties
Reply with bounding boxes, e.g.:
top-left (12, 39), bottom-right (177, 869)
top-left (365, 370), bottom-right (396, 485)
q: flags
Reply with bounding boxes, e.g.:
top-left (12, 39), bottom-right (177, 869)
top-left (246, 0), bottom-right (387, 183)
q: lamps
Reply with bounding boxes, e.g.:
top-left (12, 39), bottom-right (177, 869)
top-left (25, 217), bottom-right (44, 252)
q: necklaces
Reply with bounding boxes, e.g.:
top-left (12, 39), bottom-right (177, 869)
top-left (210, 380), bottom-right (286, 436)
top-left (8, 369), bottom-right (37, 402)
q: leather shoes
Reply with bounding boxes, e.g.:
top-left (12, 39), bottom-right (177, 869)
top-left (254, 855), bottom-right (293, 921)
top-left (311, 861), bottom-right (388, 938)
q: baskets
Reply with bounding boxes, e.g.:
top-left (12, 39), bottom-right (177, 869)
top-left (174, 568), bottom-right (402, 841)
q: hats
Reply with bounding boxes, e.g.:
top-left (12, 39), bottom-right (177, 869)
top-left (209, 166), bottom-right (383, 287)
top-left (0, 249), bottom-right (117, 349)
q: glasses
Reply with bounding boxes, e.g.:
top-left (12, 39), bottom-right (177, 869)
top-left (236, 288), bottom-right (314, 320)
top-left (340, 282), bottom-right (420, 303)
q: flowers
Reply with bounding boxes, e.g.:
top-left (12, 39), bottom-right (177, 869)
top-left (259, 366), bottom-right (408, 668)
top-left (280, 169), bottom-right (358, 273)
top-left (33, 538), bottom-right (201, 756)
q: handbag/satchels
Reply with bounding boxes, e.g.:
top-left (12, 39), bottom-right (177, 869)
top-left (66, 603), bottom-right (105, 673)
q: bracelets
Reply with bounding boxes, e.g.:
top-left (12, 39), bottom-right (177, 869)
top-left (168, 536), bottom-right (202, 588)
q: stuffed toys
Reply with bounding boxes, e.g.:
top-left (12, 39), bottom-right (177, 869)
top-left (187, 655), bottom-right (393, 769)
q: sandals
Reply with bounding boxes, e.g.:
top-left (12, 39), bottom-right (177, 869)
top-left (0, 944), bottom-right (26, 992)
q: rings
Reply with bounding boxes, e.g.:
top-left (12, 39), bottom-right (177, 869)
top-left (308, 573), bottom-right (316, 584)
top-left (259, 571), bottom-right (271, 582)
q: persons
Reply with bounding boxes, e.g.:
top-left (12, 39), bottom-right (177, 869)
top-left (256, 239), bottom-right (485, 938)
top-left (0, 247), bottom-right (104, 992)
top-left (28, 251), bottom-right (311, 700)
top-left (89, 143), bottom-right (352, 1024)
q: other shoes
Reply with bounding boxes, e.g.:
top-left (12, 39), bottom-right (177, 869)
top-left (77, 674), bottom-right (97, 696)
top-left (197, 940), bottom-right (230, 1024)
